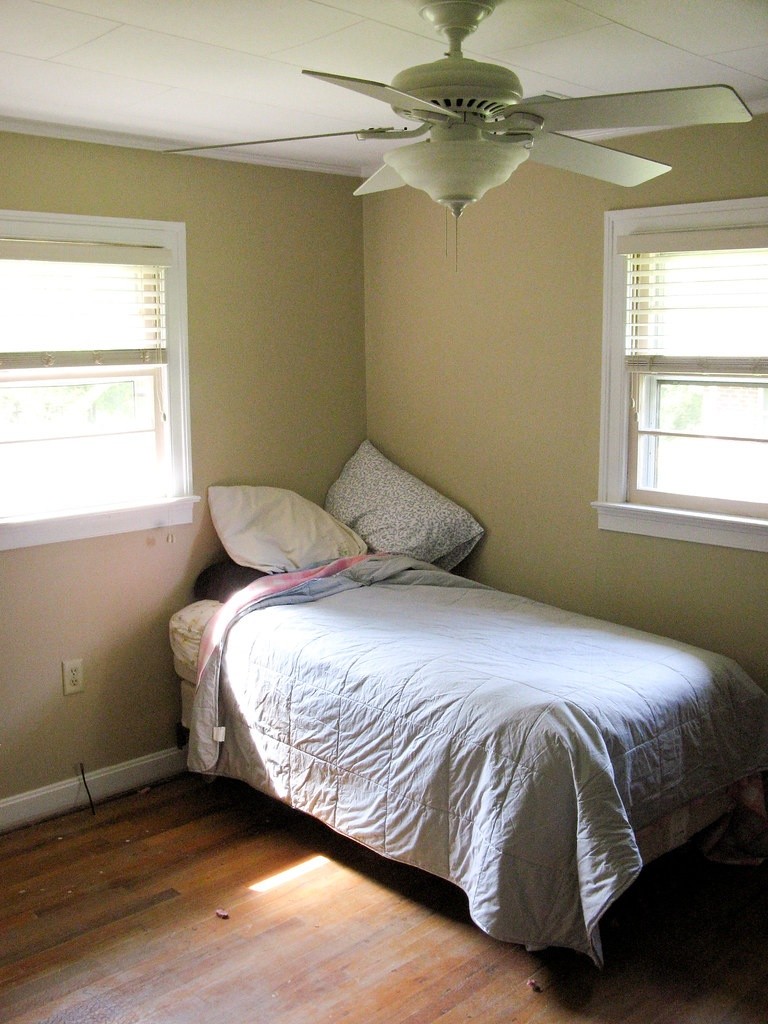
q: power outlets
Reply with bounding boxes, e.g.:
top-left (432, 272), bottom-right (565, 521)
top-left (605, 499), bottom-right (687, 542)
top-left (62, 658), bottom-right (84, 695)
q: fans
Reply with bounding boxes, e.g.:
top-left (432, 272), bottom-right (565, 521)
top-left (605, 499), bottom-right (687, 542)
top-left (164, 1), bottom-right (750, 195)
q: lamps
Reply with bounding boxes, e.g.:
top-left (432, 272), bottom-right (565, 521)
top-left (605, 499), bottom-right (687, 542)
top-left (383, 133), bottom-right (534, 219)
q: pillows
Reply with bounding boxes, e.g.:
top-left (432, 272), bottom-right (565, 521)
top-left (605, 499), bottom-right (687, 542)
top-left (323, 438), bottom-right (484, 572)
top-left (206, 484), bottom-right (365, 576)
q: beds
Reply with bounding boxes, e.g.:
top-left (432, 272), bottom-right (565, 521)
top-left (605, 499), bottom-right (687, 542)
top-left (169, 564), bottom-right (767, 968)
